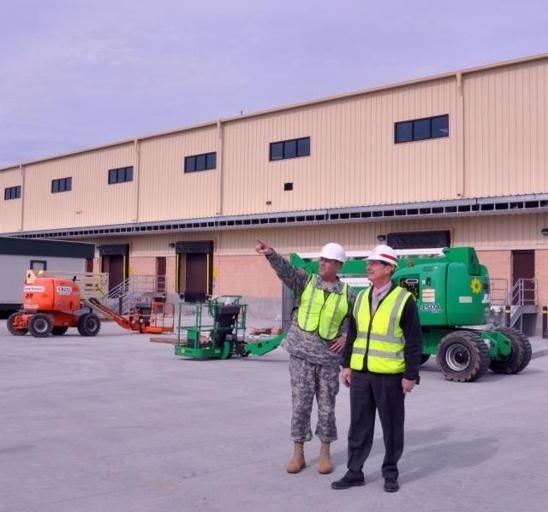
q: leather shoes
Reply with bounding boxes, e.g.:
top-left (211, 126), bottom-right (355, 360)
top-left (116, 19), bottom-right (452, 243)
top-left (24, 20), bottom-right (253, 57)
top-left (384, 478), bottom-right (398, 491)
top-left (331, 472), bottom-right (364, 489)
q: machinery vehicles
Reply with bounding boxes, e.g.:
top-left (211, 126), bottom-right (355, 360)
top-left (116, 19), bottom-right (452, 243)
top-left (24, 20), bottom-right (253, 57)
top-left (6, 271), bottom-right (174, 339)
top-left (174, 244), bottom-right (533, 382)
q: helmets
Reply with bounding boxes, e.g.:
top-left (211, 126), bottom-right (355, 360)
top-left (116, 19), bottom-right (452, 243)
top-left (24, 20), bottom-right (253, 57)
top-left (366, 244), bottom-right (398, 267)
top-left (320, 242), bottom-right (345, 264)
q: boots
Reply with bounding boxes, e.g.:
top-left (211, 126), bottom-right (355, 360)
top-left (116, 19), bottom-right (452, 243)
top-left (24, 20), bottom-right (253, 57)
top-left (319, 442), bottom-right (333, 474)
top-left (287, 442), bottom-right (305, 473)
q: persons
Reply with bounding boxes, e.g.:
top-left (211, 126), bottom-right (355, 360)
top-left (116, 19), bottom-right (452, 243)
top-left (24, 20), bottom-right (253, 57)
top-left (329, 242), bottom-right (424, 491)
top-left (253, 235), bottom-right (358, 475)
